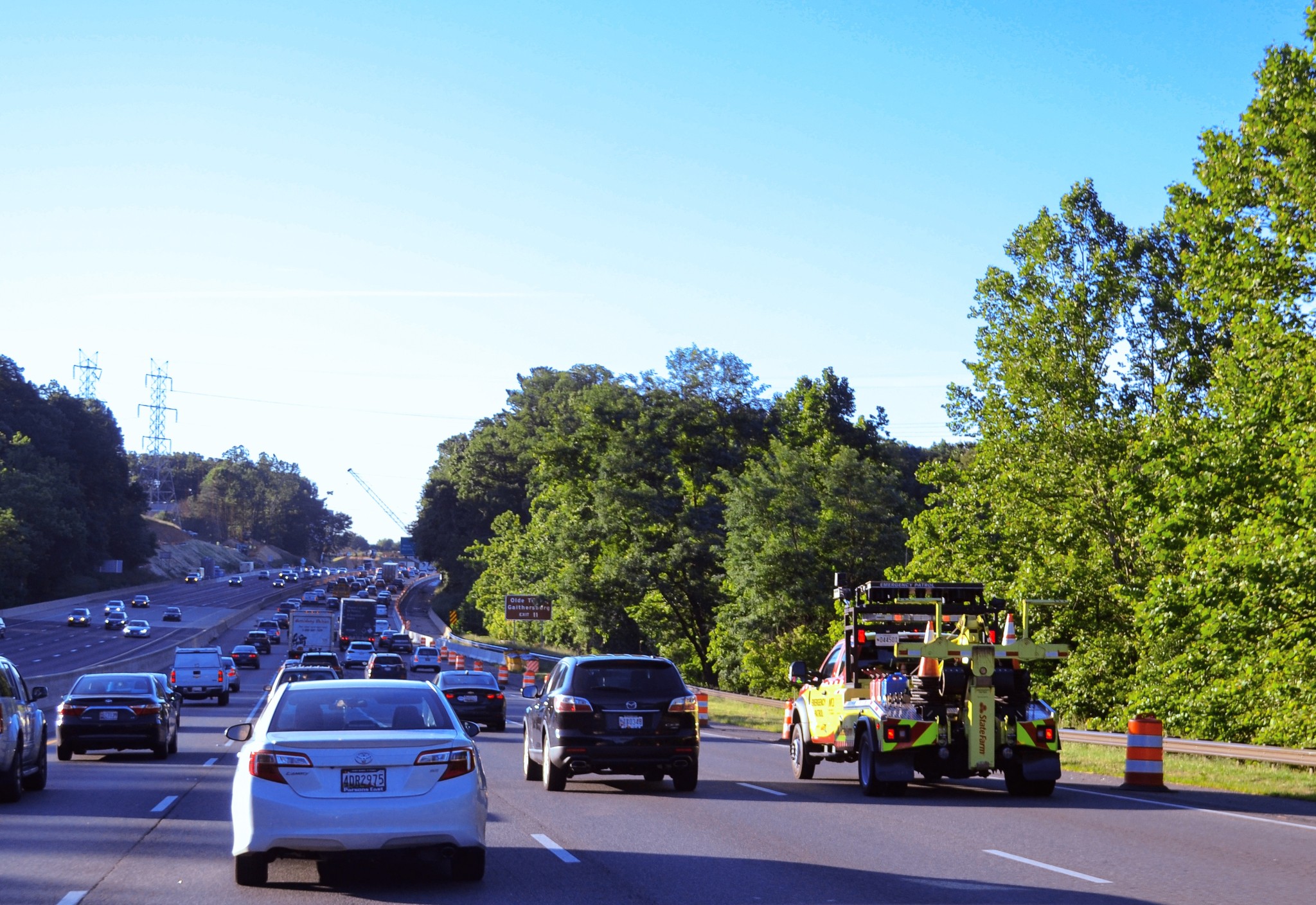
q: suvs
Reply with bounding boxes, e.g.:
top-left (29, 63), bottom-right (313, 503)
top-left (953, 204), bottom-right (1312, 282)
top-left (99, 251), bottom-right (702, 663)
top-left (254, 620), bottom-right (282, 644)
top-left (244, 630), bottom-right (273, 655)
top-left (521, 654), bottom-right (700, 793)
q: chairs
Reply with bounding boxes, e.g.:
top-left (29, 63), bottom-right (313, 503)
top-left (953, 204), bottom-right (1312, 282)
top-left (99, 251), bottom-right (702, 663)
top-left (316, 675), bottom-right (324, 680)
top-left (296, 706), bottom-right (325, 730)
top-left (392, 706), bottom-right (420, 729)
top-left (289, 674), bottom-right (298, 682)
top-left (134, 681), bottom-right (148, 689)
top-left (630, 670), bottom-right (649, 688)
top-left (90, 682), bottom-right (105, 692)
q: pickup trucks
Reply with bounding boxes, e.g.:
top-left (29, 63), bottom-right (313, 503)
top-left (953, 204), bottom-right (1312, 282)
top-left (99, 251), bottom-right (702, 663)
top-left (789, 582), bottom-right (1071, 800)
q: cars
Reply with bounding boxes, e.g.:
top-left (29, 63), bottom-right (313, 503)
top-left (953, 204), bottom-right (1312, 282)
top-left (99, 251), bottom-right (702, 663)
top-left (430, 669), bottom-right (507, 732)
top-left (162, 606), bottom-right (183, 621)
top-left (229, 645), bottom-right (261, 669)
top-left (224, 678), bottom-right (489, 887)
top-left (185, 572), bottom-right (202, 584)
top-left (68, 608), bottom-right (92, 627)
top-left (221, 656), bottom-right (240, 692)
top-left (229, 576), bottom-right (243, 586)
top-left (132, 595), bottom-right (153, 608)
top-left (0, 656), bottom-right (49, 804)
top-left (56, 673), bottom-right (184, 760)
top-left (0, 617), bottom-right (6, 638)
top-left (363, 653), bottom-right (407, 680)
top-left (258, 559), bottom-right (442, 704)
top-left (409, 646), bottom-right (442, 674)
top-left (104, 600), bottom-right (152, 638)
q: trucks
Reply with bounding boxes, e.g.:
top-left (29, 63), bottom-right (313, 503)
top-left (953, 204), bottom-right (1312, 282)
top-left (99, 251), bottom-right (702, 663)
top-left (286, 608), bottom-right (334, 659)
top-left (337, 597), bottom-right (377, 651)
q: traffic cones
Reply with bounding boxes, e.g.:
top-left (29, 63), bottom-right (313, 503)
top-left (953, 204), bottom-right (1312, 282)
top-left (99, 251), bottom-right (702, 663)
top-left (917, 620), bottom-right (940, 677)
top-left (1000, 614), bottom-right (1015, 645)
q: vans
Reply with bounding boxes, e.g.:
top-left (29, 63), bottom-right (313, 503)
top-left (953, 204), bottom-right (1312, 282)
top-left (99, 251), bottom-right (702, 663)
top-left (169, 645), bottom-right (231, 707)
